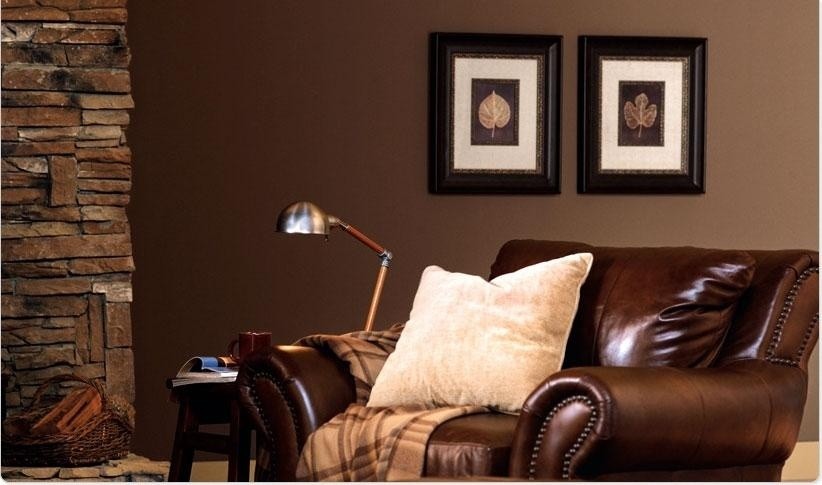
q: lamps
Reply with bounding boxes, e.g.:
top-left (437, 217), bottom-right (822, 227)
top-left (272, 199), bottom-right (393, 332)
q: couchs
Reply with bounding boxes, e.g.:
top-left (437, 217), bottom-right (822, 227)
top-left (234, 236), bottom-right (819, 480)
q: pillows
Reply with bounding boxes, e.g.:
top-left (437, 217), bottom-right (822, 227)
top-left (364, 252), bottom-right (595, 411)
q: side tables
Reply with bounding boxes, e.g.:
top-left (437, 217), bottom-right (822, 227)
top-left (165, 379), bottom-right (259, 482)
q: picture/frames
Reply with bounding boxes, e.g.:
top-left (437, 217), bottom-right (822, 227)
top-left (426, 29), bottom-right (563, 196)
top-left (576, 32), bottom-right (707, 199)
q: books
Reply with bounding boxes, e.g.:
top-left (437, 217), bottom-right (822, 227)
top-left (172, 356), bottom-right (238, 387)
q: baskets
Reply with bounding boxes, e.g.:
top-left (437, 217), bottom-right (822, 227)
top-left (2, 374), bottom-right (135, 468)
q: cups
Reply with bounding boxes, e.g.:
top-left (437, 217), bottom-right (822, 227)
top-left (228, 332), bottom-right (271, 367)
